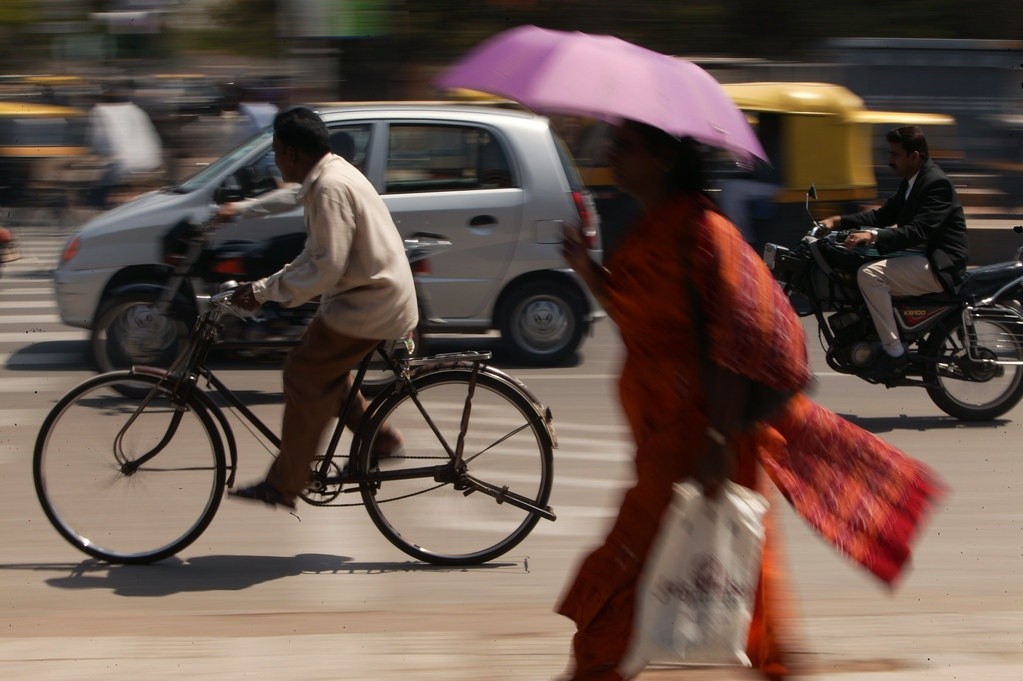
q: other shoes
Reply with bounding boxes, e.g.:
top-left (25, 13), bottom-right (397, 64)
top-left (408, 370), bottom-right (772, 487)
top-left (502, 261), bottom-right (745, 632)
top-left (861, 349), bottom-right (908, 378)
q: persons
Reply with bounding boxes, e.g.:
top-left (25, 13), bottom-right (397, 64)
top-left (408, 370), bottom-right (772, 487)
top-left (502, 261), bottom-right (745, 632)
top-left (88, 94), bottom-right (161, 209)
top-left (552, 122), bottom-right (947, 681)
top-left (228, 107), bottom-right (418, 511)
top-left (278, 131), bottom-right (360, 187)
top-left (817, 126), bottom-right (969, 376)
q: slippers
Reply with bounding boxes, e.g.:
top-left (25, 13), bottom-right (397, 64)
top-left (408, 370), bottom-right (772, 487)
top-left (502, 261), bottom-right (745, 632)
top-left (227, 481), bottom-right (295, 511)
top-left (342, 425), bottom-right (406, 476)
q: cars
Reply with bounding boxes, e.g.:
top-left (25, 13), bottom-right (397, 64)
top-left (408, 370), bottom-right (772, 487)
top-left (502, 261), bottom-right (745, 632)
top-left (49, 100), bottom-right (608, 366)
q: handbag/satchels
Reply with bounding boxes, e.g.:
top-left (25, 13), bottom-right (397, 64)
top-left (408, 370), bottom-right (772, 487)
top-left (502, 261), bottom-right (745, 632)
top-left (619, 477), bottom-right (769, 676)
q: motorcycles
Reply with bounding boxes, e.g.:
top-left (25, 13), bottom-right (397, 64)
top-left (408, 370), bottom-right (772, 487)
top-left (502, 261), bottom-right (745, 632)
top-left (0, 58), bottom-right (268, 211)
top-left (431, 79), bottom-right (954, 281)
top-left (765, 183), bottom-right (1022, 424)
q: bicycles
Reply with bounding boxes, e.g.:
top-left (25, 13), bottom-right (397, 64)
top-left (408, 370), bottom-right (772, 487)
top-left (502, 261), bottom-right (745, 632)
top-left (31, 278), bottom-right (561, 570)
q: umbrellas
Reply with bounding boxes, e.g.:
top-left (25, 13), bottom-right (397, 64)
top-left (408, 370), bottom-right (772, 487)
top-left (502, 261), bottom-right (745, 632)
top-left (437, 27), bottom-right (771, 222)
